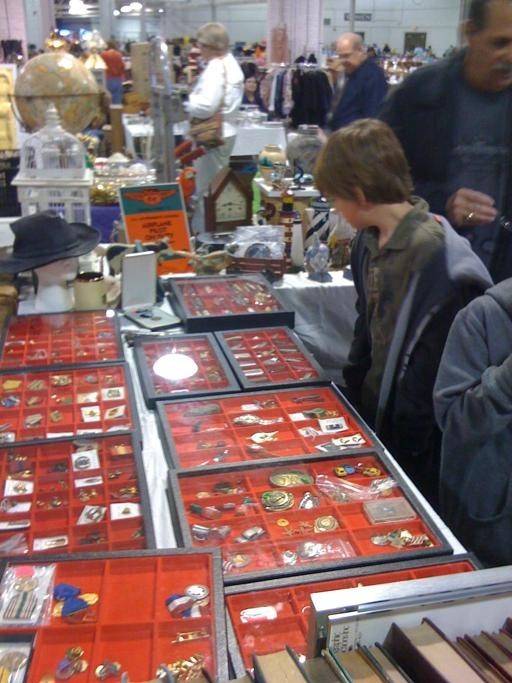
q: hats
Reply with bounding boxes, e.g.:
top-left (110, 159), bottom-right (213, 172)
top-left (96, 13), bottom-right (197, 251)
top-left (1, 209), bottom-right (102, 274)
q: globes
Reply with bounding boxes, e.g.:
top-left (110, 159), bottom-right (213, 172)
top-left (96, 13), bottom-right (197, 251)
top-left (8, 52), bottom-right (101, 135)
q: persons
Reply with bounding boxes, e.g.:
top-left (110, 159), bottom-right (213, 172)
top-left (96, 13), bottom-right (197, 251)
top-left (179, 20), bottom-right (245, 122)
top-left (101, 38), bottom-right (125, 104)
top-left (241, 71), bottom-right (269, 116)
top-left (374, 0), bottom-right (512, 288)
top-left (327, 31), bottom-right (389, 132)
top-left (313, 116), bottom-right (494, 497)
top-left (430, 275), bottom-right (511, 568)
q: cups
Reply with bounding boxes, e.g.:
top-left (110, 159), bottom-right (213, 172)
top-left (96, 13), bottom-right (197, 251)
top-left (75, 272), bottom-right (108, 312)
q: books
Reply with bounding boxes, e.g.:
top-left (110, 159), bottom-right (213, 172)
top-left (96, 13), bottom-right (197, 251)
top-left (178, 614), bottom-right (512, 683)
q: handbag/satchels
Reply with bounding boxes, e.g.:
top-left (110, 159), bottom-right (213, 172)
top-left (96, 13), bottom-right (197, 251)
top-left (189, 111), bottom-right (224, 150)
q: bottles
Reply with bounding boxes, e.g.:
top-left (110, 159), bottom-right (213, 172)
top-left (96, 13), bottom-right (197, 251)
top-left (258, 144), bottom-right (286, 186)
top-left (290, 218), bottom-right (304, 266)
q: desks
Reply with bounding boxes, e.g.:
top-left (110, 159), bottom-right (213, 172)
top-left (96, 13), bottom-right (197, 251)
top-left (0, 96), bottom-right (478, 683)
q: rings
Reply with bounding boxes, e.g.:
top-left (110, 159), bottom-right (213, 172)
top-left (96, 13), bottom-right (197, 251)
top-left (464, 208), bottom-right (475, 223)
